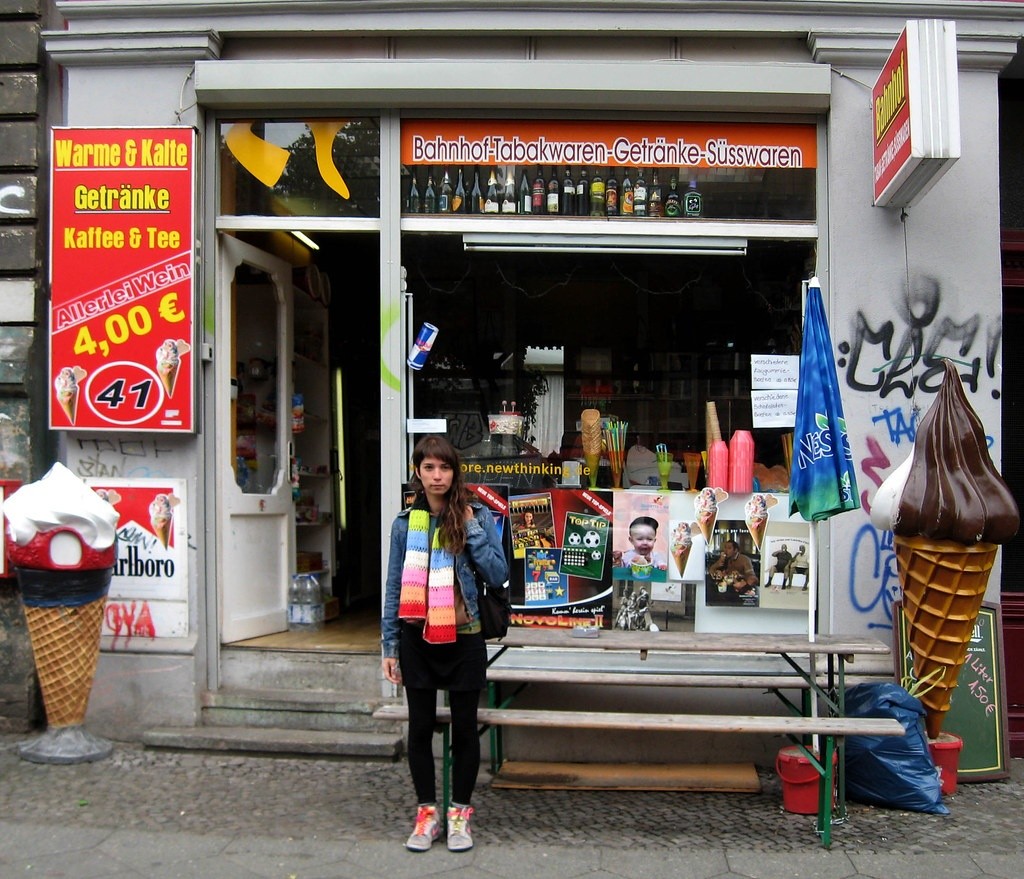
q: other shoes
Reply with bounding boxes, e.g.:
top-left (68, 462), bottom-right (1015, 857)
top-left (787, 585), bottom-right (792, 589)
top-left (802, 584), bottom-right (807, 591)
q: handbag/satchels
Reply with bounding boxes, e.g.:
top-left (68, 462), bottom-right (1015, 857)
top-left (465, 543), bottom-right (513, 643)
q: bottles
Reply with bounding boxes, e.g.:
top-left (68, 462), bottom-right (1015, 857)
top-left (605, 167), bottom-right (620, 216)
top-left (470, 165), bottom-right (485, 214)
top-left (561, 165), bottom-right (576, 216)
top-left (532, 165), bottom-right (546, 215)
top-left (683, 180), bottom-right (704, 218)
top-left (648, 167), bottom-right (663, 217)
top-left (452, 166), bottom-right (469, 214)
top-left (620, 167), bottom-right (633, 216)
top-left (546, 165), bottom-right (561, 215)
top-left (634, 168), bottom-right (648, 216)
top-left (502, 166), bottom-right (518, 215)
top-left (518, 165), bottom-right (532, 215)
top-left (423, 164), bottom-right (438, 212)
top-left (589, 165), bottom-right (605, 216)
top-left (485, 166), bottom-right (500, 213)
top-left (664, 174), bottom-right (683, 217)
top-left (576, 165), bottom-right (589, 216)
top-left (438, 165), bottom-right (452, 213)
top-left (287, 574), bottom-right (324, 634)
top-left (406, 165), bottom-right (422, 213)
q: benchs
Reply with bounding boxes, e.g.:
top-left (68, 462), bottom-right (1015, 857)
top-left (483, 666), bottom-right (859, 768)
top-left (372, 703), bottom-right (905, 850)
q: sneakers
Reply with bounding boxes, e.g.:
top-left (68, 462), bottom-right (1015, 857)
top-left (406, 802), bottom-right (441, 850)
top-left (446, 806), bottom-right (473, 850)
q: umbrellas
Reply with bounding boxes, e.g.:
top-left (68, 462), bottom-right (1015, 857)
top-left (786, 278), bottom-right (860, 646)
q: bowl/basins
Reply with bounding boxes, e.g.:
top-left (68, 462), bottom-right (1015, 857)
top-left (631, 555), bottom-right (653, 579)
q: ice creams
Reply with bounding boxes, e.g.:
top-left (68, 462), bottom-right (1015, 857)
top-left (155, 339), bottom-right (191, 398)
top-left (55, 366), bottom-right (87, 426)
top-left (149, 493), bottom-right (180, 551)
top-left (870, 358), bottom-right (1019, 742)
top-left (669, 488), bottom-right (778, 578)
top-left (1, 462), bottom-right (120, 726)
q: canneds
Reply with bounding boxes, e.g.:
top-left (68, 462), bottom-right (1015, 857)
top-left (407, 322), bottom-right (440, 370)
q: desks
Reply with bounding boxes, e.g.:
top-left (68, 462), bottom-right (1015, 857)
top-left (443, 626), bottom-right (890, 818)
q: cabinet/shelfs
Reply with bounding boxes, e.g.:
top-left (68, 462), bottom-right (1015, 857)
top-left (565, 353), bottom-right (699, 433)
top-left (229, 261), bottom-right (338, 621)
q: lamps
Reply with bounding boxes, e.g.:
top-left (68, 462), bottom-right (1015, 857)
top-left (463, 232), bottom-right (748, 257)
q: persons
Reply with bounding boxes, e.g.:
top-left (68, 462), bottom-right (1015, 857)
top-left (710, 539), bottom-right (755, 588)
top-left (764, 544), bottom-right (810, 590)
top-left (613, 516), bottom-right (667, 570)
top-left (612, 586), bottom-right (649, 631)
top-left (512, 508), bottom-right (536, 533)
top-left (382, 434), bottom-right (511, 851)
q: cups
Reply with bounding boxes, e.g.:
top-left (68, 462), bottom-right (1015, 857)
top-left (608, 450), bottom-right (624, 490)
top-left (684, 453), bottom-right (702, 492)
top-left (585, 453), bottom-right (602, 489)
top-left (656, 452), bottom-right (673, 491)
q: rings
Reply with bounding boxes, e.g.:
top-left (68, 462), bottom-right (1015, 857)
top-left (391, 667), bottom-right (395, 670)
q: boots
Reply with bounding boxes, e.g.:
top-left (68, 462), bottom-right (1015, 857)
top-left (781, 578), bottom-right (787, 589)
top-left (764, 577), bottom-right (772, 587)
